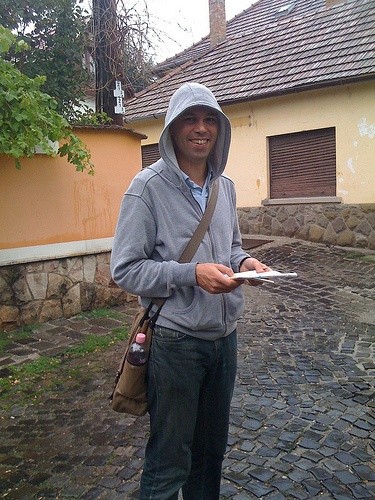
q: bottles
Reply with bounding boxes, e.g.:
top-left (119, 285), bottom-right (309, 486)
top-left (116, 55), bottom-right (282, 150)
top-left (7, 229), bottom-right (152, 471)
top-left (126, 333), bottom-right (148, 366)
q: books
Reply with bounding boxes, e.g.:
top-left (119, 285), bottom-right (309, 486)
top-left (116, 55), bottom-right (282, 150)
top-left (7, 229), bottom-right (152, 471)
top-left (226, 269), bottom-right (298, 279)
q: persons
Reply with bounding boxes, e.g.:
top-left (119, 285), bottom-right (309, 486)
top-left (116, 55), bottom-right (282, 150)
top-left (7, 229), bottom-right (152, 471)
top-left (110, 82), bottom-right (277, 500)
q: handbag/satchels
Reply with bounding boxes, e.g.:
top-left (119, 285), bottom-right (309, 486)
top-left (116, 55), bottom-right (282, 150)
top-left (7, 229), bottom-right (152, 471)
top-left (109, 308), bottom-right (153, 416)
